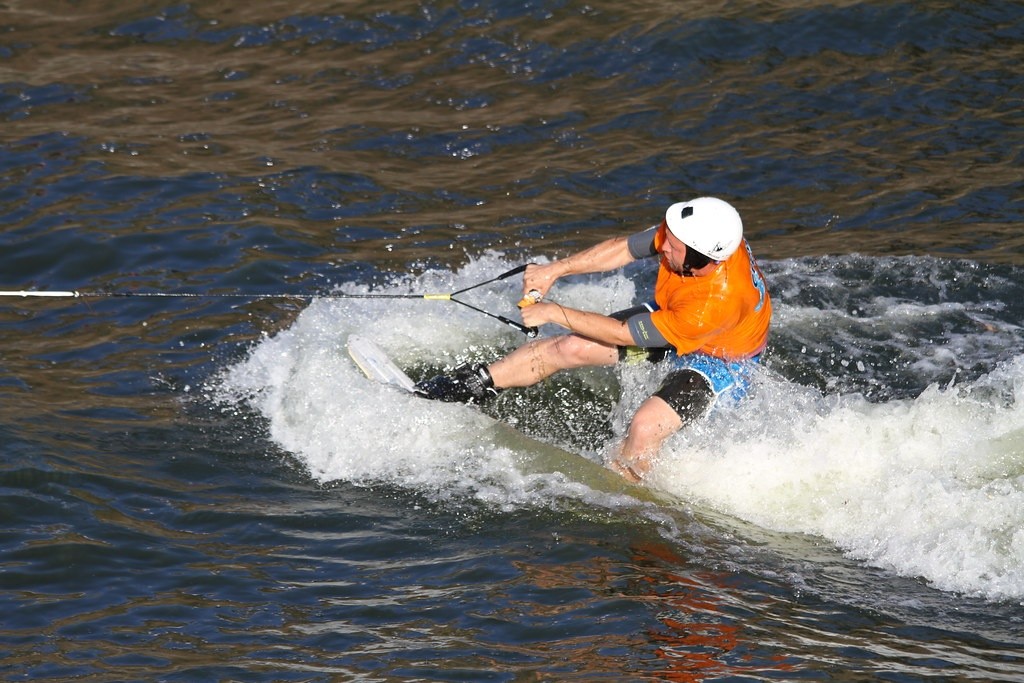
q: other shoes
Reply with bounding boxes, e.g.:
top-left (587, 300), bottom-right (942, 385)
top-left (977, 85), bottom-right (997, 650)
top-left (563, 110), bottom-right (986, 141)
top-left (419, 364), bottom-right (500, 407)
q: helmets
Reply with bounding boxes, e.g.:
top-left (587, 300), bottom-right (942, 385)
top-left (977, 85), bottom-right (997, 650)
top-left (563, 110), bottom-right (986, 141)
top-left (666, 195), bottom-right (743, 262)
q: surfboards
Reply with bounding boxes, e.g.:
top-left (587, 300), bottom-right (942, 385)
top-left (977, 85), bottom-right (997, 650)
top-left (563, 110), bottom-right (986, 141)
top-left (347, 332), bottom-right (831, 561)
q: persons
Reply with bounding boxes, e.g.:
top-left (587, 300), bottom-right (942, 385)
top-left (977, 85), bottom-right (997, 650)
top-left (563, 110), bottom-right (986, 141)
top-left (414, 196), bottom-right (772, 462)
